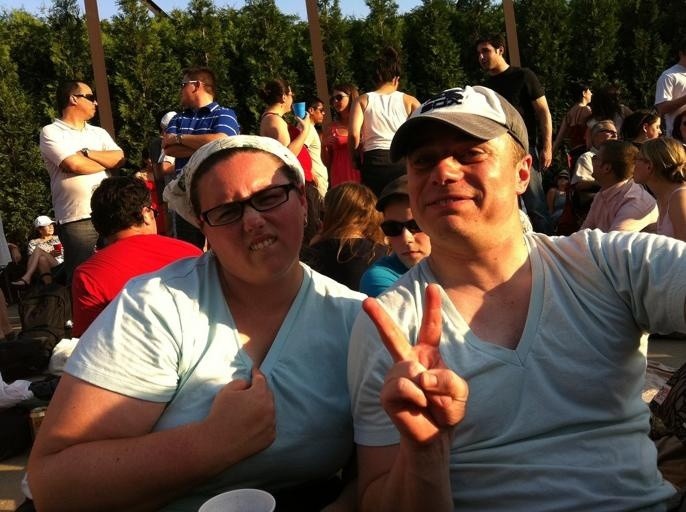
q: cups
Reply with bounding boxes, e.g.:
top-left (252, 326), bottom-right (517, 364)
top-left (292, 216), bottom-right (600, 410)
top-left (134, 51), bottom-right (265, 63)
top-left (290, 101), bottom-right (305, 120)
top-left (52, 243), bottom-right (61, 255)
top-left (195, 487), bottom-right (275, 512)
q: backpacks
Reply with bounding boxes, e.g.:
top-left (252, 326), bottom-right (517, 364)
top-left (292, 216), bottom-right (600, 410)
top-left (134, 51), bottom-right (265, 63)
top-left (19, 284), bottom-right (72, 343)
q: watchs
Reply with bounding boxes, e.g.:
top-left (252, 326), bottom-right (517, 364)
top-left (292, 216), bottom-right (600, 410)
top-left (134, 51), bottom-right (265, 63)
top-left (82, 148), bottom-right (88, 157)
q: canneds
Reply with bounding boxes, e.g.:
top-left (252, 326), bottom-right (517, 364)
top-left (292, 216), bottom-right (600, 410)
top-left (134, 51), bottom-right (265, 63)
top-left (29, 406), bottom-right (47, 439)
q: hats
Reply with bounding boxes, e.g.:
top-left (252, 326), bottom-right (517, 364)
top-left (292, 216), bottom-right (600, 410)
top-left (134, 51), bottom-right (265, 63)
top-left (375, 175), bottom-right (409, 210)
top-left (390, 86), bottom-right (529, 164)
top-left (35, 216), bottom-right (56, 227)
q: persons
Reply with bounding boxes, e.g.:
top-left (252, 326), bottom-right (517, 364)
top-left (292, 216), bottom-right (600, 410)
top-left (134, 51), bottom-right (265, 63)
top-left (346, 85), bottom-right (685, 512)
top-left (654, 42), bottom-right (685, 138)
top-left (542, 80), bottom-right (685, 242)
top-left (10, 215), bottom-right (65, 286)
top-left (16, 135), bottom-right (369, 512)
top-left (476, 39), bottom-right (555, 235)
top-left (1, 228), bottom-right (12, 338)
top-left (257, 46), bottom-right (432, 296)
top-left (134, 66), bottom-right (240, 251)
top-left (28, 175), bottom-right (203, 401)
top-left (40, 81), bottom-right (124, 320)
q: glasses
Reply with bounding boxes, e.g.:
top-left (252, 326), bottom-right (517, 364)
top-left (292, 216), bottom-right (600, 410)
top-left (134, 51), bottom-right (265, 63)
top-left (329, 94), bottom-right (347, 102)
top-left (201, 184), bottom-right (298, 227)
top-left (380, 220), bottom-right (420, 237)
top-left (180, 80), bottom-right (198, 86)
top-left (74, 94), bottom-right (94, 102)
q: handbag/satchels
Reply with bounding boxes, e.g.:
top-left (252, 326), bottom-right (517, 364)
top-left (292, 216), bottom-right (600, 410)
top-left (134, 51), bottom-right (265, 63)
top-left (0, 336), bottom-right (48, 384)
